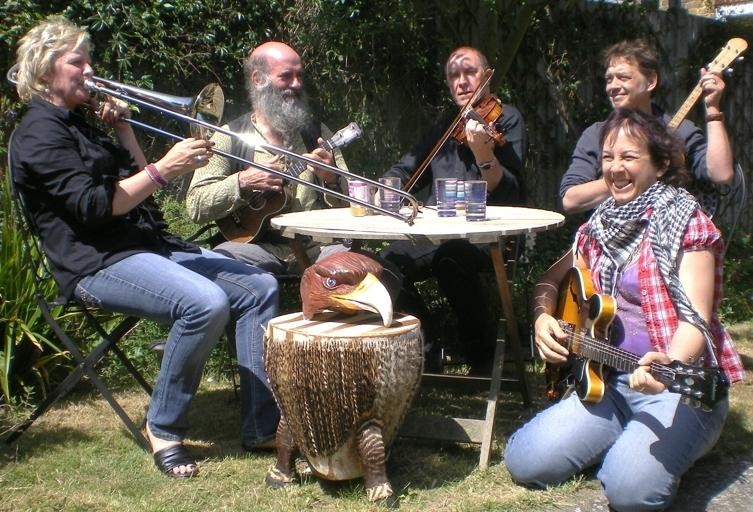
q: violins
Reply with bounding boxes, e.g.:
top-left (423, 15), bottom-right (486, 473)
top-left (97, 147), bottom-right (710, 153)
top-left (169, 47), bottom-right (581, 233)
top-left (452, 94), bottom-right (507, 147)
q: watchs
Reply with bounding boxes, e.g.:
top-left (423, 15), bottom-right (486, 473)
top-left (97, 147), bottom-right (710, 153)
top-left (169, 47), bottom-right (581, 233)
top-left (478, 155), bottom-right (497, 172)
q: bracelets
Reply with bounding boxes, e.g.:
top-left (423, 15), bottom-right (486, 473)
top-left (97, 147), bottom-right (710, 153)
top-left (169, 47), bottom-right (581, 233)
top-left (705, 111), bottom-right (726, 123)
top-left (328, 174), bottom-right (343, 190)
top-left (143, 161), bottom-right (169, 189)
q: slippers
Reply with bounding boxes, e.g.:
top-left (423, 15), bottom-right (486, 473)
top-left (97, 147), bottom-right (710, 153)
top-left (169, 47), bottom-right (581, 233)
top-left (147, 434), bottom-right (197, 477)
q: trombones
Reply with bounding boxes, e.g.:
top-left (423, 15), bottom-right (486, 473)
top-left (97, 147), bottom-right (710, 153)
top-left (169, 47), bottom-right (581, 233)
top-left (6, 64), bottom-right (418, 222)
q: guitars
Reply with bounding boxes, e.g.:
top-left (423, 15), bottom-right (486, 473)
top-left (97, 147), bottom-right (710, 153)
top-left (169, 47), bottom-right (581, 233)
top-left (215, 122), bottom-right (362, 244)
top-left (546, 267), bottom-right (727, 413)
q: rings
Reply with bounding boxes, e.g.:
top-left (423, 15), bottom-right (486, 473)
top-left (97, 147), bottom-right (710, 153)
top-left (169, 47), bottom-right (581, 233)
top-left (641, 385), bottom-right (645, 393)
top-left (195, 153), bottom-right (205, 165)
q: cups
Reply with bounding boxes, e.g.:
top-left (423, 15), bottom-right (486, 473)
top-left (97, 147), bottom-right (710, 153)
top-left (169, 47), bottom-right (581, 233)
top-left (377, 176), bottom-right (401, 217)
top-left (434, 178), bottom-right (487, 222)
top-left (347, 181), bottom-right (374, 217)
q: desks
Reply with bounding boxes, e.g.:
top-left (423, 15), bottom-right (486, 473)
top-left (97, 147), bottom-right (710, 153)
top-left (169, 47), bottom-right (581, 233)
top-left (266, 196), bottom-right (578, 476)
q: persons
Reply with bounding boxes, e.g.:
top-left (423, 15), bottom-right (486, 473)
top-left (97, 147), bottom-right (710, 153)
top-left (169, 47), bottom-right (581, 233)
top-left (183, 39), bottom-right (403, 309)
top-left (10, 18), bottom-right (288, 479)
top-left (373, 45), bottom-right (527, 374)
top-left (504, 105), bottom-right (743, 508)
top-left (559, 38), bottom-right (734, 218)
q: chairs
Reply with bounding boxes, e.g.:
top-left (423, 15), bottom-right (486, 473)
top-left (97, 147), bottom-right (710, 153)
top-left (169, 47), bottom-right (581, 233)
top-left (0, 129), bottom-right (157, 461)
top-left (672, 159), bottom-right (748, 259)
